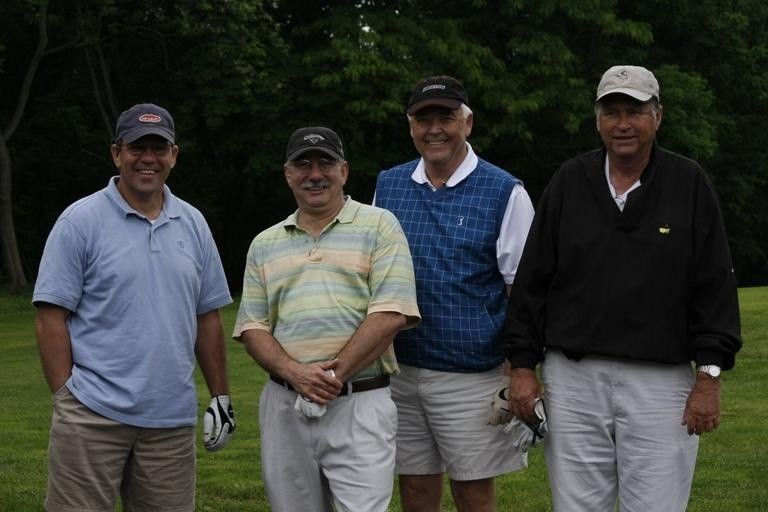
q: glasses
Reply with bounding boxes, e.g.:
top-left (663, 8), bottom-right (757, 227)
top-left (118, 144), bottom-right (172, 156)
top-left (290, 156), bottom-right (340, 170)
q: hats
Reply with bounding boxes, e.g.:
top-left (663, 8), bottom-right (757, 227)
top-left (595, 65), bottom-right (659, 104)
top-left (406, 75), bottom-right (472, 116)
top-left (115, 103), bottom-right (176, 146)
top-left (286, 127), bottom-right (345, 161)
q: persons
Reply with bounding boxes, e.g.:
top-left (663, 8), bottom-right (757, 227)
top-left (368, 70), bottom-right (539, 512)
top-left (499, 62), bottom-right (743, 510)
top-left (30, 102), bottom-right (236, 511)
top-left (229, 124), bottom-right (424, 512)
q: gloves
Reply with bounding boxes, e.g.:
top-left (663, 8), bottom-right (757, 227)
top-left (202, 395), bottom-right (236, 454)
top-left (294, 369), bottom-right (336, 425)
top-left (485, 384), bottom-right (549, 453)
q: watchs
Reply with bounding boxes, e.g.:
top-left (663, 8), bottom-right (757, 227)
top-left (694, 363), bottom-right (724, 380)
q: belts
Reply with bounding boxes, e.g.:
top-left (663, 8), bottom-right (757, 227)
top-left (269, 372), bottom-right (391, 396)
